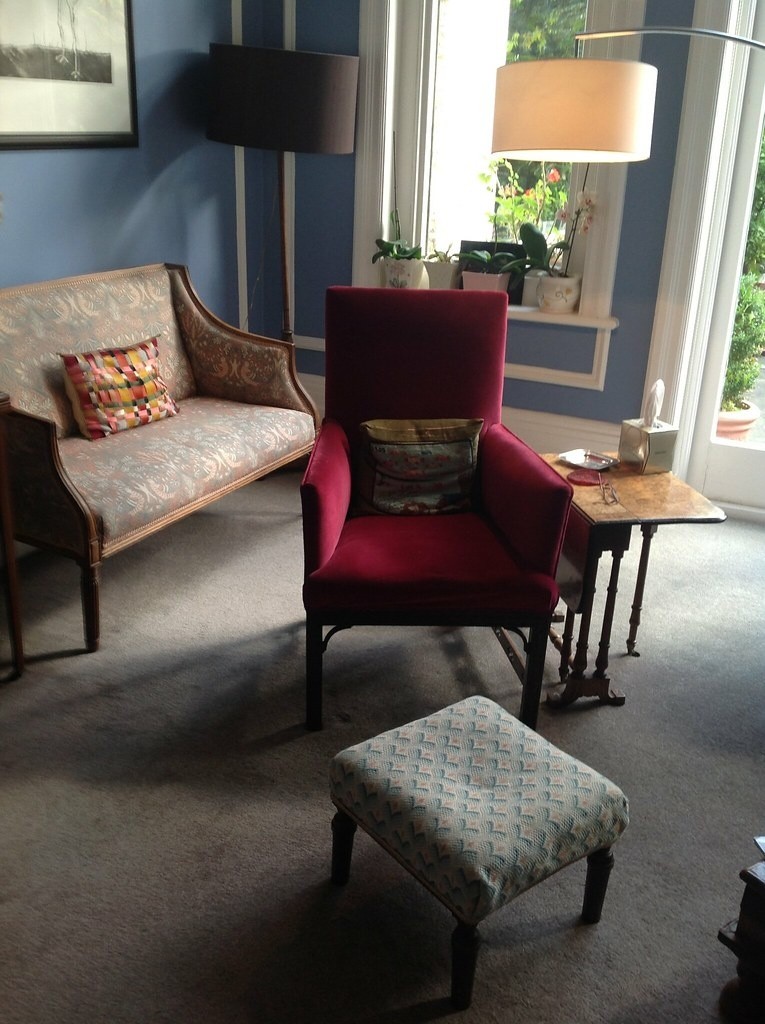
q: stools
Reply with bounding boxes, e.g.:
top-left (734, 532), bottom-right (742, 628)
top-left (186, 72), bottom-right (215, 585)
top-left (320, 694), bottom-right (632, 1011)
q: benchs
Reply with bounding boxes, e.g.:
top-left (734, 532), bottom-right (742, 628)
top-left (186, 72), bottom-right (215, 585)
top-left (0, 262), bottom-right (321, 653)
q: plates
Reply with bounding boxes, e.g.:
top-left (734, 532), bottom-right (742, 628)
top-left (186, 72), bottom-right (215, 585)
top-left (559, 448), bottom-right (621, 470)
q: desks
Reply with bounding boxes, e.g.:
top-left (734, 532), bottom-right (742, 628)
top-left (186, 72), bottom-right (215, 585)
top-left (533, 450), bottom-right (726, 707)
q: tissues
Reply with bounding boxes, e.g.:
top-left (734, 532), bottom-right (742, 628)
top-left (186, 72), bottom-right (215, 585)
top-left (616, 378), bottom-right (679, 475)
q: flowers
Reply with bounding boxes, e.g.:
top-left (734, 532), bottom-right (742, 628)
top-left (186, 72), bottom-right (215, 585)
top-left (479, 159), bottom-right (566, 246)
top-left (542, 191), bottom-right (594, 278)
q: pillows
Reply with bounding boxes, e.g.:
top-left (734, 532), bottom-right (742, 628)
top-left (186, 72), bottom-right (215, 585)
top-left (347, 418), bottom-right (484, 517)
top-left (53, 332), bottom-right (183, 439)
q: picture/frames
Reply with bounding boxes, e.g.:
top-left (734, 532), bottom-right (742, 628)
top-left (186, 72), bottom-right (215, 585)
top-left (0, 0), bottom-right (139, 151)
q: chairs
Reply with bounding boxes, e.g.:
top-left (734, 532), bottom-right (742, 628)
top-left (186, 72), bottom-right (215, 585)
top-left (298, 283), bottom-right (574, 729)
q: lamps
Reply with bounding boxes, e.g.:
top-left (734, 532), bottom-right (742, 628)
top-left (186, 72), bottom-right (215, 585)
top-left (198, 43), bottom-right (358, 334)
top-left (490, 25), bottom-right (765, 164)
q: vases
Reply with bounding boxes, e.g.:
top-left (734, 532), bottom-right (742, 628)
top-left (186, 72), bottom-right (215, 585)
top-left (537, 276), bottom-right (582, 316)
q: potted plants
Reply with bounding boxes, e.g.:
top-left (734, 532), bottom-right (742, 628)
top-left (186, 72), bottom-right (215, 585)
top-left (715, 139), bottom-right (765, 444)
top-left (371, 237), bottom-right (429, 290)
top-left (499, 224), bottom-right (570, 307)
top-left (423, 244), bottom-right (462, 290)
top-left (455, 249), bottom-right (522, 295)
